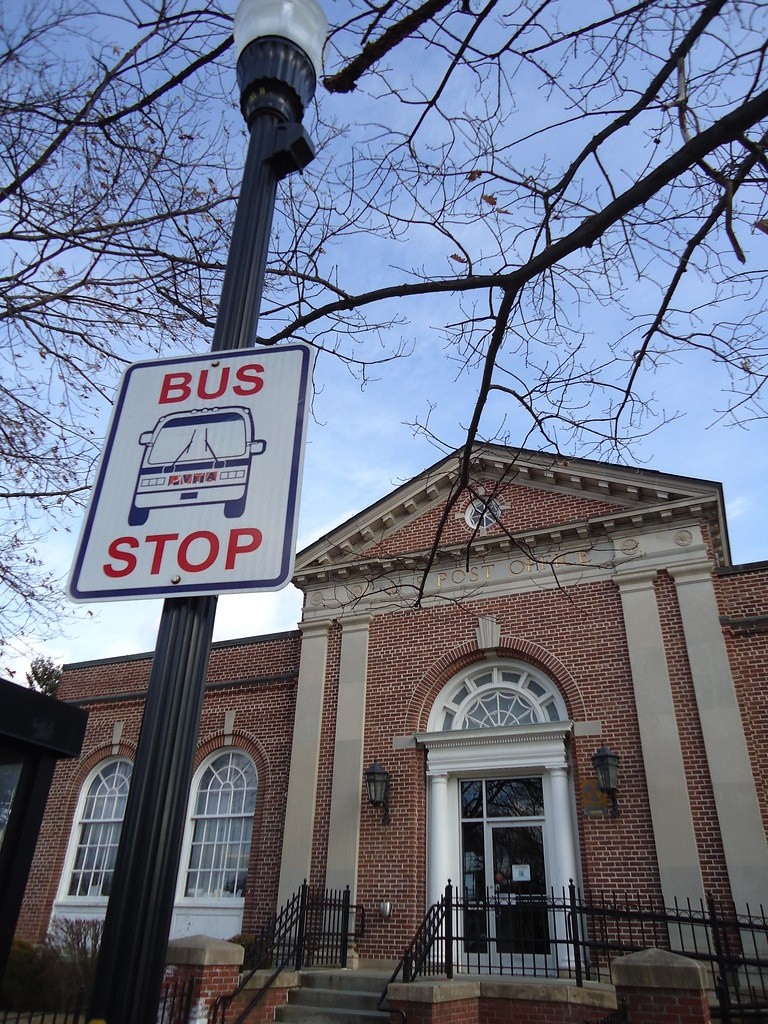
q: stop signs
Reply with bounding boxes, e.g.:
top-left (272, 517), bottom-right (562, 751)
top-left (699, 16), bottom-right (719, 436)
top-left (67, 340), bottom-right (311, 603)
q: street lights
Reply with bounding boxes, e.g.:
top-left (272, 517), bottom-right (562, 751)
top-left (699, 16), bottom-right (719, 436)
top-left (80, 0), bottom-right (330, 1022)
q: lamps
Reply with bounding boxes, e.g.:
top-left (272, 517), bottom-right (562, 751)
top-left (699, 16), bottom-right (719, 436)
top-left (363, 759), bottom-right (392, 824)
top-left (592, 740), bottom-right (623, 817)
